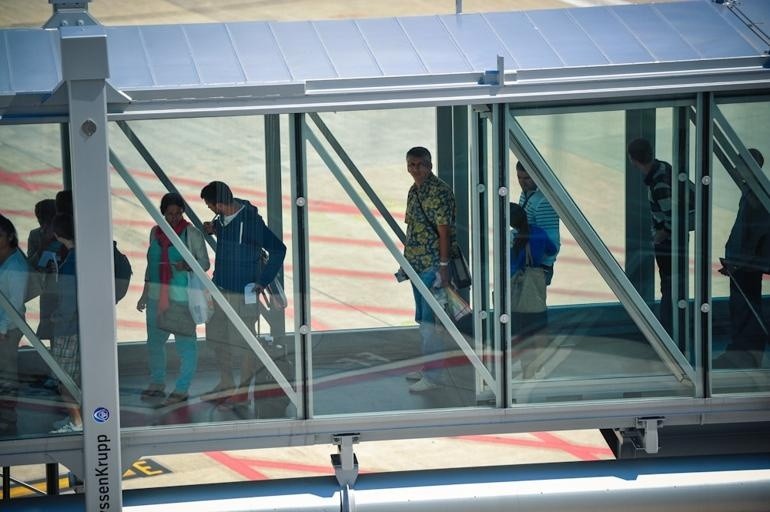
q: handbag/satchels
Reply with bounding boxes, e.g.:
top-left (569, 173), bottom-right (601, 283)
top-left (114, 240), bottom-right (132, 302)
top-left (449, 245), bottom-right (471, 288)
top-left (157, 303), bottom-right (196, 336)
top-left (511, 267), bottom-right (547, 314)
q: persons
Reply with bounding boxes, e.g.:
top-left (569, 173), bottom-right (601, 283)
top-left (628, 137), bottom-right (695, 354)
top-left (718, 148), bottom-right (769, 352)
top-left (136, 192), bottom-right (210, 407)
top-left (511, 161), bottom-right (562, 284)
top-left (398, 147), bottom-right (458, 393)
top-left (200, 180), bottom-right (287, 410)
top-left (1, 190), bottom-right (83, 437)
top-left (511, 203), bottom-right (558, 381)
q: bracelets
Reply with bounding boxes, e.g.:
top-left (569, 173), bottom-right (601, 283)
top-left (145, 279), bottom-right (149, 282)
top-left (440, 262), bottom-right (448, 267)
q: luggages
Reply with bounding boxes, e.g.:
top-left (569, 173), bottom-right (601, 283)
top-left (254, 290), bottom-right (294, 418)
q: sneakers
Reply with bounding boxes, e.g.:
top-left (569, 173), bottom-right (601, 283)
top-left (201, 384), bottom-right (250, 410)
top-left (142, 387), bottom-right (186, 404)
top-left (1, 377), bottom-right (82, 436)
top-left (405, 372), bottom-right (442, 393)
